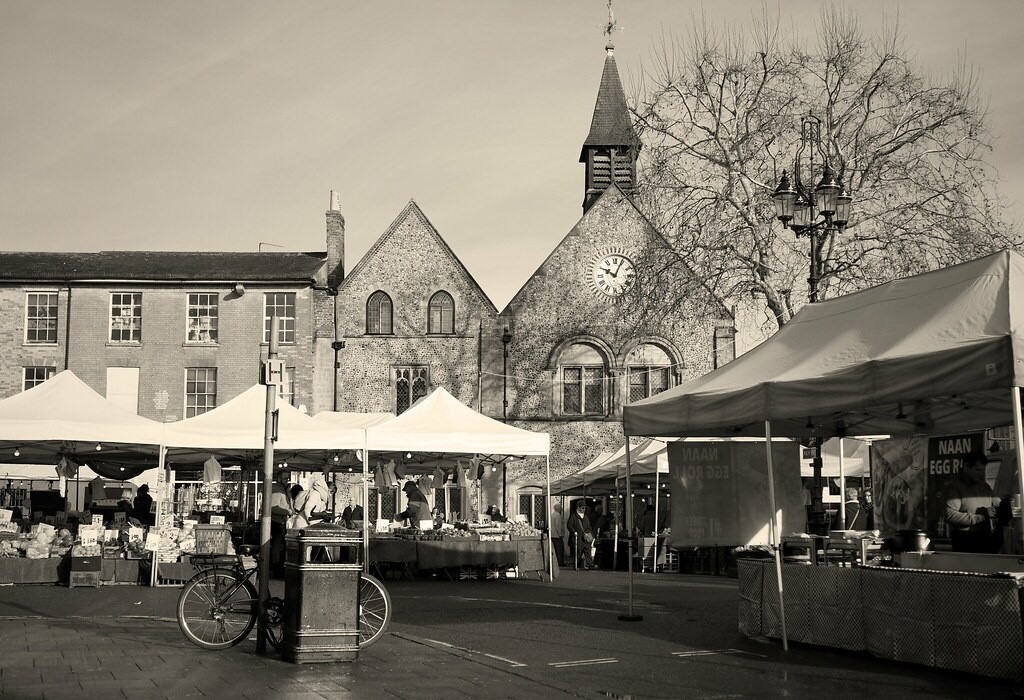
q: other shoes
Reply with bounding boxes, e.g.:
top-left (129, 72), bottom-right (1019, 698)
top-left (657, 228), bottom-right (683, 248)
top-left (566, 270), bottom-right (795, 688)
top-left (573, 565), bottom-right (580, 571)
top-left (558, 562), bottom-right (568, 567)
top-left (585, 564), bottom-right (598, 569)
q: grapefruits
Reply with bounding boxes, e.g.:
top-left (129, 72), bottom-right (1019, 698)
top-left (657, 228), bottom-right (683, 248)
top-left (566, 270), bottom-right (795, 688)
top-left (72, 544), bottom-right (101, 557)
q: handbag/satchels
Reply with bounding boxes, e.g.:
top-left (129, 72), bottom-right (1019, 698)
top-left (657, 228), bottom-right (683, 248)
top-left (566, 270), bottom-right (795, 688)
top-left (584, 532), bottom-right (594, 542)
top-left (591, 538), bottom-right (596, 557)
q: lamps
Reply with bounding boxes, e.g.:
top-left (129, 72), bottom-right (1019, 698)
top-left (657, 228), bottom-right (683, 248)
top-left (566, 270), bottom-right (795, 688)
top-left (235, 284), bottom-right (245, 296)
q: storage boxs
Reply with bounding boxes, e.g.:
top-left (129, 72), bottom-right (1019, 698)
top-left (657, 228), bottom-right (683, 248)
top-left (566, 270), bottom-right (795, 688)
top-left (193, 524), bottom-right (232, 556)
top-left (637, 537), bottom-right (655, 557)
top-left (69, 540), bottom-right (103, 588)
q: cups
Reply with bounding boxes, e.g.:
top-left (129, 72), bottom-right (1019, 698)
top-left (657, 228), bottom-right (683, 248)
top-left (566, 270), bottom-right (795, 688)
top-left (1014, 494), bottom-right (1020, 507)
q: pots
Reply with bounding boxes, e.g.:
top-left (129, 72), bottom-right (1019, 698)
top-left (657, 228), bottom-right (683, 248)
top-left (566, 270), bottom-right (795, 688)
top-left (883, 528), bottom-right (930, 561)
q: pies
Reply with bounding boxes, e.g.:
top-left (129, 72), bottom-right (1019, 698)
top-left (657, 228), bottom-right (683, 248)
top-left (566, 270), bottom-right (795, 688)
top-left (870, 447), bottom-right (925, 531)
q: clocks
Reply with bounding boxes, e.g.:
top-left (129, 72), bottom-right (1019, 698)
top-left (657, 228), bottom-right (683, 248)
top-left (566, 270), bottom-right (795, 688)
top-left (593, 253), bottom-right (637, 298)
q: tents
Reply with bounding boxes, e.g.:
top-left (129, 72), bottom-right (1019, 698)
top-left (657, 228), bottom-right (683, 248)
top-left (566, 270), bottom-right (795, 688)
top-left (0, 370), bottom-right (553, 583)
top-left (617, 248), bottom-right (1024, 621)
top-left (542, 436), bottom-right (890, 573)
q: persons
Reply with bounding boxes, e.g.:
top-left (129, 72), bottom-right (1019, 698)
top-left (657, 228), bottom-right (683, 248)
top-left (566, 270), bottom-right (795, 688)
top-left (567, 500), bottom-right (598, 571)
top-left (395, 481), bottom-right (437, 531)
top-left (642, 504), bottom-right (655, 537)
top-left (271, 469), bottom-right (295, 582)
top-left (835, 488), bottom-right (867, 532)
top-left (552, 504), bottom-right (568, 567)
top-left (863, 488), bottom-right (873, 530)
top-left (943, 450), bottom-right (1002, 554)
top-left (585, 504), bottom-right (614, 538)
top-left (286, 473), bottom-right (338, 561)
top-left (132, 484), bottom-right (153, 532)
top-left (342, 497), bottom-right (363, 530)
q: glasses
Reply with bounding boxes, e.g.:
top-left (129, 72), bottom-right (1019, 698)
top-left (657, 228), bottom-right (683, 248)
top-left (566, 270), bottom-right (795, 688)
top-left (581, 507), bottom-right (587, 509)
top-left (280, 477), bottom-right (289, 480)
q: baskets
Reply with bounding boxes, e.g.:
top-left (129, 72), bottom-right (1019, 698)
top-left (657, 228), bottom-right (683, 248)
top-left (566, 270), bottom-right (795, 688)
top-left (193, 522), bottom-right (232, 555)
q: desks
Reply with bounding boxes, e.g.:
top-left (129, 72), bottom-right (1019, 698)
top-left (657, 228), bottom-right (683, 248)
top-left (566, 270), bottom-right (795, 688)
top-left (156, 563), bottom-right (257, 587)
top-left (509, 534), bottom-right (559, 582)
top-left (0, 557), bottom-right (139, 586)
top-left (360, 539), bottom-right (518, 580)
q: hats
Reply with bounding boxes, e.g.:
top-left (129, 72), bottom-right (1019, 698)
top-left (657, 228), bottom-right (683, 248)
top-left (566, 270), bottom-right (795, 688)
top-left (647, 505), bottom-right (655, 510)
top-left (576, 500), bottom-right (587, 506)
top-left (402, 481), bottom-right (416, 491)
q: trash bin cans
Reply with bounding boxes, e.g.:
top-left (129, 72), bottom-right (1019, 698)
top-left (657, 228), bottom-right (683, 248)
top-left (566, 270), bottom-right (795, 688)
top-left (285, 522), bottom-right (362, 664)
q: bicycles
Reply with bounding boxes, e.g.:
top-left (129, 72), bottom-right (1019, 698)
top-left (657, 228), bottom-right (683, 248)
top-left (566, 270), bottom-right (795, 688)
top-left (176, 505), bottom-right (391, 649)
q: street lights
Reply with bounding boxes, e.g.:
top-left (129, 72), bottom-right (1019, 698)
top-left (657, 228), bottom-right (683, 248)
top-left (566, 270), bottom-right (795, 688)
top-left (325, 275), bottom-right (346, 524)
top-left (500, 334), bottom-right (512, 520)
top-left (771, 108), bottom-right (852, 536)
